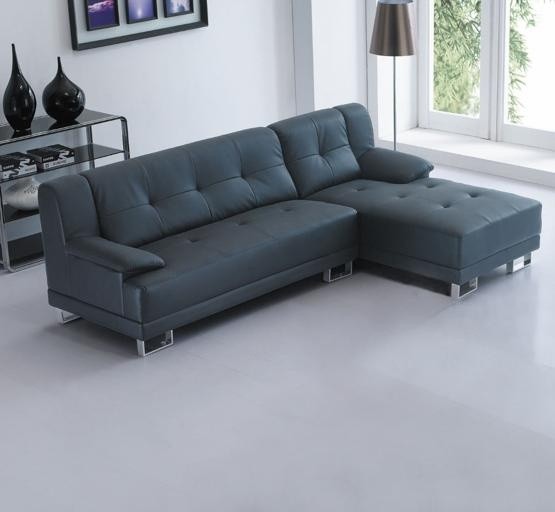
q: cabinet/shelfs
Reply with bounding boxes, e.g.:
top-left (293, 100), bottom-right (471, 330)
top-left (0, 108), bottom-right (131, 274)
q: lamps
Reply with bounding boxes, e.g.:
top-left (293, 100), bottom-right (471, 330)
top-left (368, 0), bottom-right (416, 152)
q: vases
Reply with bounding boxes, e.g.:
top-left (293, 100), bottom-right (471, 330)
top-left (3, 43), bottom-right (85, 130)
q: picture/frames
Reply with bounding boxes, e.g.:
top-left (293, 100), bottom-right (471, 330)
top-left (68, 0), bottom-right (209, 51)
